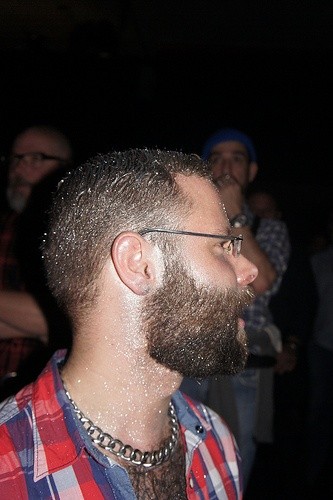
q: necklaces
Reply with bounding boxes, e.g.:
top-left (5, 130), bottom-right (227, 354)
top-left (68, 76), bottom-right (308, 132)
top-left (58, 378), bottom-right (177, 466)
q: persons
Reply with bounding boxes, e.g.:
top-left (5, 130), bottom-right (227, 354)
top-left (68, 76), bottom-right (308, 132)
top-left (248, 191), bottom-right (318, 500)
top-left (1, 147), bottom-right (258, 500)
top-left (300, 210), bottom-right (333, 500)
top-left (180, 129), bottom-right (290, 500)
top-left (1, 113), bottom-right (83, 401)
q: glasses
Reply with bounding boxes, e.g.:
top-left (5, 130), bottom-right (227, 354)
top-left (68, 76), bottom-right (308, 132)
top-left (8, 151), bottom-right (68, 166)
top-left (140, 224), bottom-right (248, 258)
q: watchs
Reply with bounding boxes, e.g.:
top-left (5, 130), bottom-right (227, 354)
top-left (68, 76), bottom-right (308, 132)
top-left (230, 213), bottom-right (248, 229)
top-left (283, 340), bottom-right (302, 355)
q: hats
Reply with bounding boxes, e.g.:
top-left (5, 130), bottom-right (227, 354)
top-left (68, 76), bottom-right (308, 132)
top-left (200, 127), bottom-right (256, 165)
